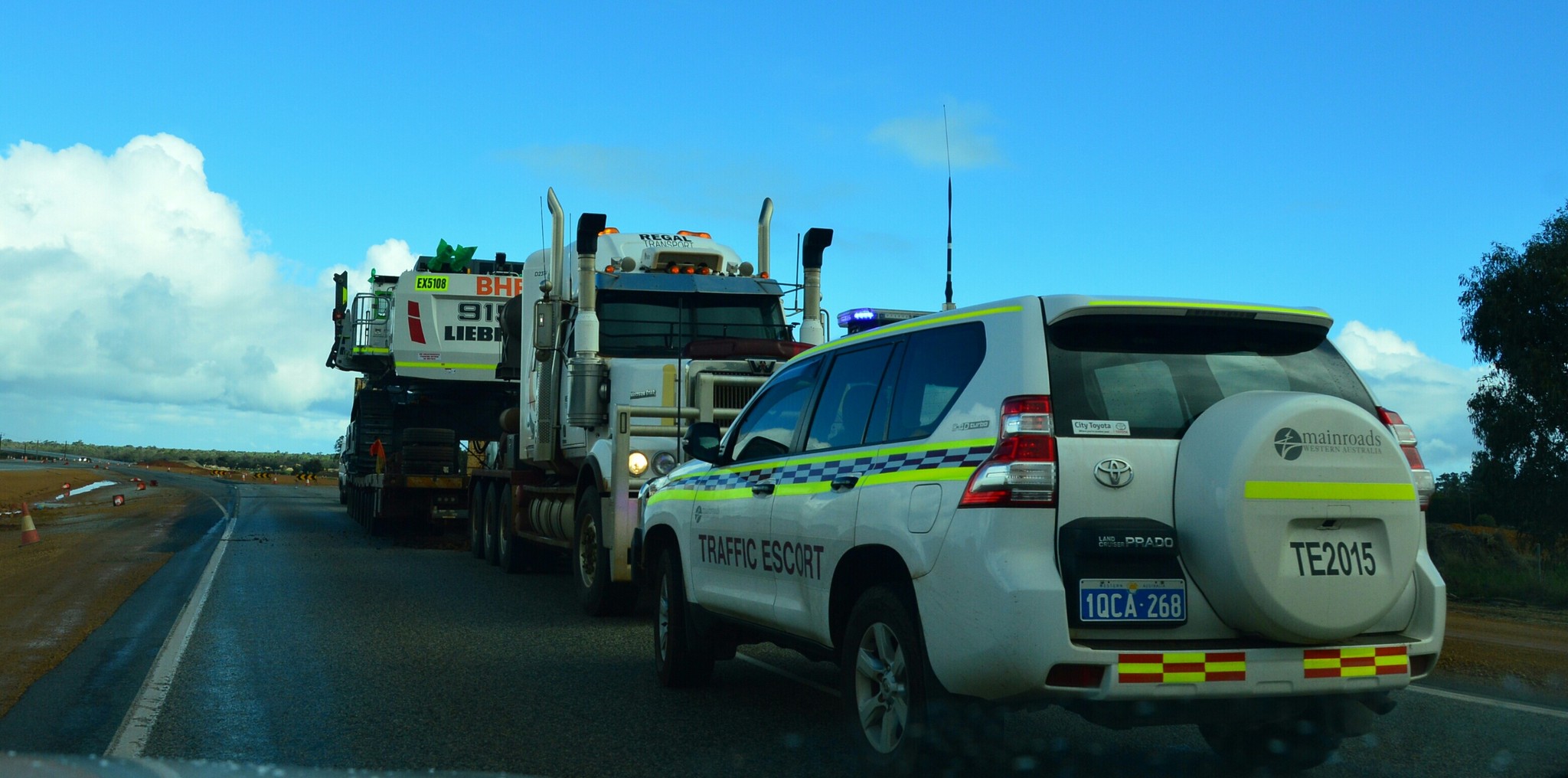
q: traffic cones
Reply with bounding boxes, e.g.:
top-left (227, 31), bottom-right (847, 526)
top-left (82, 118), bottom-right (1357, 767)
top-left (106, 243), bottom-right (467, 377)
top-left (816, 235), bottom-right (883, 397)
top-left (23, 456), bottom-right (194, 476)
top-left (129, 477), bottom-right (144, 483)
top-left (241, 473), bottom-right (246, 481)
top-left (112, 494), bottom-right (125, 506)
top-left (274, 473), bottom-right (278, 484)
top-left (149, 480), bottom-right (158, 486)
top-left (305, 478), bottom-right (311, 487)
top-left (136, 483), bottom-right (146, 491)
top-left (18, 502), bottom-right (43, 548)
top-left (61, 482), bottom-right (71, 490)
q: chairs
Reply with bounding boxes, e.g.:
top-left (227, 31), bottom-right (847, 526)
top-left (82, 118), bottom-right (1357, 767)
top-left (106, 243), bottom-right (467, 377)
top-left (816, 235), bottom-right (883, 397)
top-left (826, 383), bottom-right (878, 447)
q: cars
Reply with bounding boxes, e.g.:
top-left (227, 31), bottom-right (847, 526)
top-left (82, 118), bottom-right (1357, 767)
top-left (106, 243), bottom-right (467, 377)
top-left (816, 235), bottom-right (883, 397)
top-left (78, 457), bottom-right (93, 463)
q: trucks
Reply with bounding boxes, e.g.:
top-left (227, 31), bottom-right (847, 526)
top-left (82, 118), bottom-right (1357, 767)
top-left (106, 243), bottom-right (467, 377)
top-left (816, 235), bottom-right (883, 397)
top-left (324, 183), bottom-right (834, 619)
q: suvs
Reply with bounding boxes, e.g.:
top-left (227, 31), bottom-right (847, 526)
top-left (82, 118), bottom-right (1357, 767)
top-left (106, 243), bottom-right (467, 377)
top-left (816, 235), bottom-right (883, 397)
top-left (628, 289), bottom-right (1452, 778)
